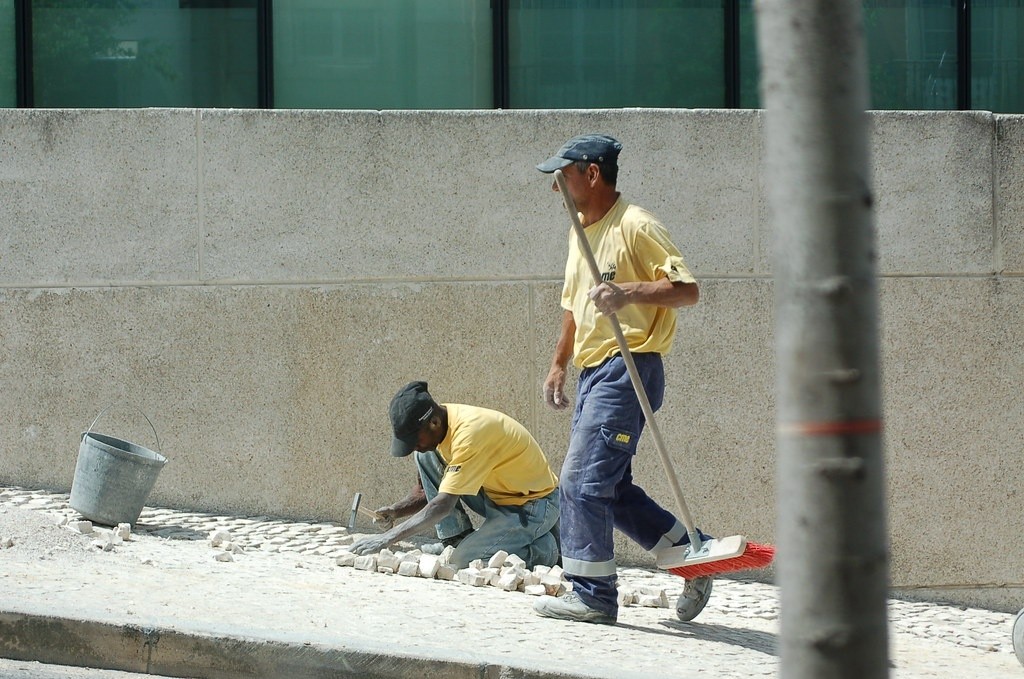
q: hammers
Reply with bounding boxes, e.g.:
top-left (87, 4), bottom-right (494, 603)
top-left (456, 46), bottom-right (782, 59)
top-left (348, 493), bottom-right (396, 528)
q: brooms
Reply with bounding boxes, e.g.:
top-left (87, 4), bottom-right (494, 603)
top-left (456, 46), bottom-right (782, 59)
top-left (553, 169), bottom-right (776, 581)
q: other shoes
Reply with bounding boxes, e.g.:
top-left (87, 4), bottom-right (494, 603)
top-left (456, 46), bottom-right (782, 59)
top-left (549, 526), bottom-right (563, 567)
top-left (437, 526), bottom-right (475, 548)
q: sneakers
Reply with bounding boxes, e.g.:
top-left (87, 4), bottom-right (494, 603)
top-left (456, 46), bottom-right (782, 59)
top-left (676, 571), bottom-right (714, 621)
top-left (533, 592), bottom-right (618, 625)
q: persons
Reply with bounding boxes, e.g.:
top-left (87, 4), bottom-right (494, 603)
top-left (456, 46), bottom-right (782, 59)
top-left (532, 133), bottom-right (713, 625)
top-left (347, 380), bottom-right (560, 569)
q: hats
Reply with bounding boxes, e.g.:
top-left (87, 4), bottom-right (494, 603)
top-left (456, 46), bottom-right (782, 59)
top-left (536, 133), bottom-right (623, 173)
top-left (389, 381), bottom-right (435, 457)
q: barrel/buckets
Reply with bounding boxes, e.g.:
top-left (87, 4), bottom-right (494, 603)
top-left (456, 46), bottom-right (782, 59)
top-left (69, 401), bottom-right (169, 528)
